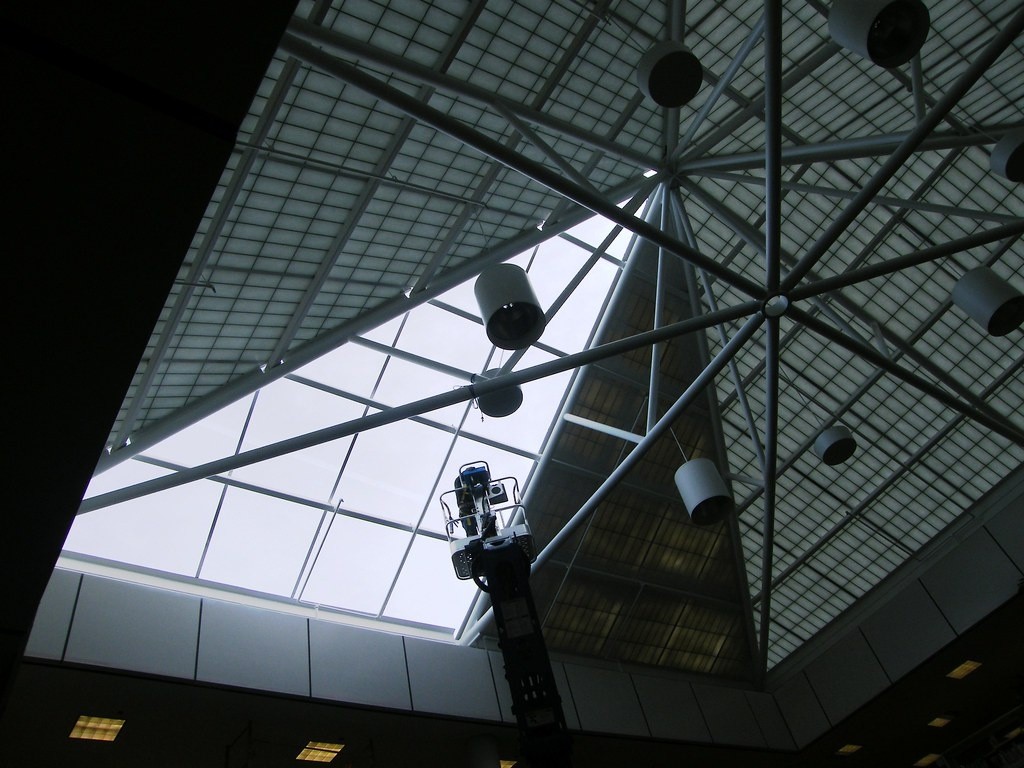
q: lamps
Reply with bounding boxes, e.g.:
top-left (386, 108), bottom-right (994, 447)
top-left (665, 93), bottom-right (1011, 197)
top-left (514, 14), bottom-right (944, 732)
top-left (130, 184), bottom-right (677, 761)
top-left (803, 0), bottom-right (930, 67)
top-left (468, 205), bottom-right (546, 349)
top-left (603, 11), bottom-right (705, 110)
top-left (670, 426), bottom-right (735, 527)
top-left (898, 221), bottom-right (1024, 338)
top-left (776, 363), bottom-right (856, 466)
top-left (478, 348), bottom-right (524, 418)
top-left (898, 74), bottom-right (1023, 183)
